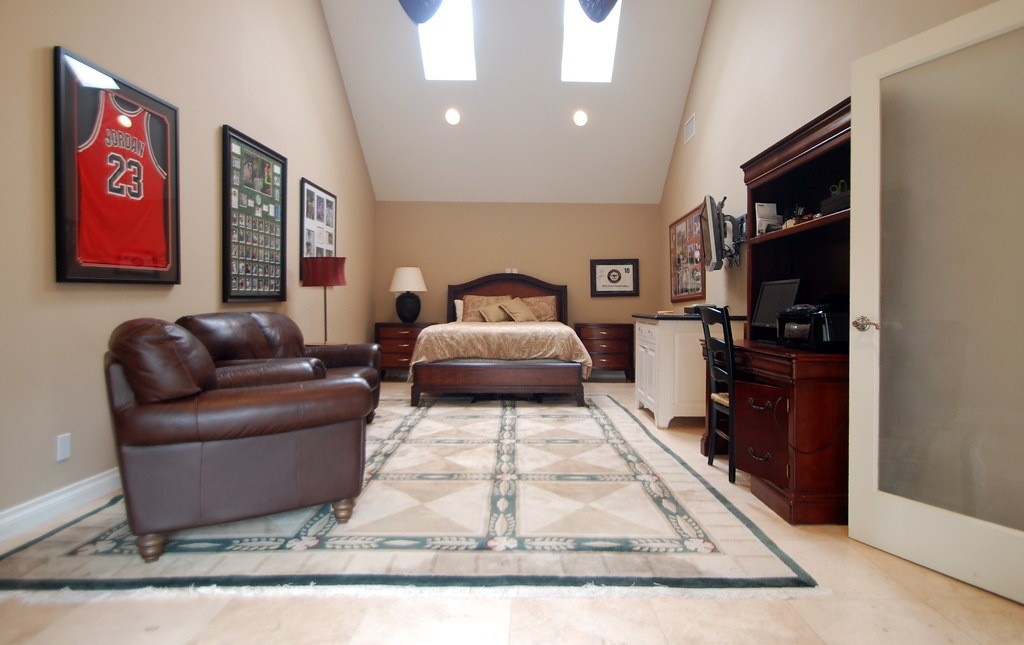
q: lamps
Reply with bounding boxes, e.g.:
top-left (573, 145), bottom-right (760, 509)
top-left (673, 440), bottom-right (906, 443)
top-left (389, 267), bottom-right (427, 323)
top-left (300, 257), bottom-right (347, 343)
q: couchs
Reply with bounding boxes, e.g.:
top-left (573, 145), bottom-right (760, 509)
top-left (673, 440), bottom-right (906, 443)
top-left (105, 311), bottom-right (384, 563)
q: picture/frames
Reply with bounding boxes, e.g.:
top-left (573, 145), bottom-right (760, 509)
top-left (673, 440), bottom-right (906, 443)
top-left (668, 202), bottom-right (706, 302)
top-left (299, 177), bottom-right (337, 279)
top-left (222, 124), bottom-right (287, 302)
top-left (51, 45), bottom-right (182, 285)
top-left (589, 259), bottom-right (640, 297)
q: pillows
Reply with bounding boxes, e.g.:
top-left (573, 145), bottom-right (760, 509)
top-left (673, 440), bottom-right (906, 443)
top-left (521, 296), bottom-right (557, 322)
top-left (463, 294), bottom-right (512, 321)
top-left (500, 297), bottom-right (538, 322)
top-left (477, 303), bottom-right (510, 323)
top-left (454, 300), bottom-right (464, 322)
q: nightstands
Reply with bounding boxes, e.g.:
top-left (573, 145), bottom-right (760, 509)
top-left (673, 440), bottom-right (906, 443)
top-left (374, 322), bottom-right (440, 379)
top-left (574, 323), bottom-right (635, 383)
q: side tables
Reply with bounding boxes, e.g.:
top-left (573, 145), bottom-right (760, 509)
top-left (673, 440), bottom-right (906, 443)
top-left (305, 342), bottom-right (346, 346)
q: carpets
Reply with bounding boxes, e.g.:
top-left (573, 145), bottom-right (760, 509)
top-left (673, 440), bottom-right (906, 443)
top-left (0, 394), bottom-right (832, 601)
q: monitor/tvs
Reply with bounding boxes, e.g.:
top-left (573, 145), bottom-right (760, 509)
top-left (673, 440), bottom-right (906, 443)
top-left (700, 194), bottom-right (741, 271)
top-left (751, 279), bottom-right (802, 344)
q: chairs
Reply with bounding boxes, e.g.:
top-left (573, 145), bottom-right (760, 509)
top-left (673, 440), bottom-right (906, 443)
top-left (698, 305), bottom-right (737, 483)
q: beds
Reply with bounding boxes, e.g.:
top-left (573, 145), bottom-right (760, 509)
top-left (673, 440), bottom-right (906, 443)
top-left (408, 273), bottom-right (593, 407)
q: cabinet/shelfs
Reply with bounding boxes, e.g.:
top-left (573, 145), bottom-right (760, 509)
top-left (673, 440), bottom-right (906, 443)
top-left (631, 313), bottom-right (747, 429)
top-left (701, 97), bottom-right (851, 526)
